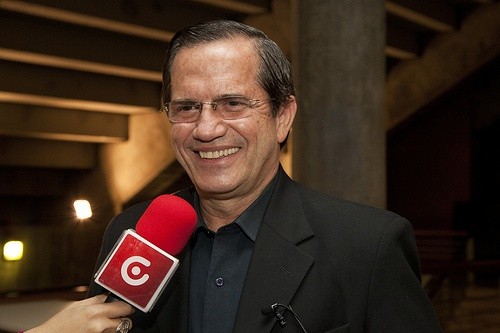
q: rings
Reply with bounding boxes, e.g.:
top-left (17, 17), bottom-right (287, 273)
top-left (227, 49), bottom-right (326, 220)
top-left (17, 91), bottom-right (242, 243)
top-left (116, 317), bottom-right (131, 333)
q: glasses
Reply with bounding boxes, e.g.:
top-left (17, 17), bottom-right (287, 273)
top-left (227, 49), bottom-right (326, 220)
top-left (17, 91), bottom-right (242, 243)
top-left (159, 95), bottom-right (275, 123)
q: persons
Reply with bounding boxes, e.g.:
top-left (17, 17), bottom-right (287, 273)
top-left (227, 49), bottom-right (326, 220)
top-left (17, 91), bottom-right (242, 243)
top-left (81, 17), bottom-right (443, 333)
top-left (15, 292), bottom-right (139, 333)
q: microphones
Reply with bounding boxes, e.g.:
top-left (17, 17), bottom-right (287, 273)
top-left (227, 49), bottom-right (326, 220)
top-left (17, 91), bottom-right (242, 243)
top-left (93, 195), bottom-right (198, 314)
top-left (260, 303), bottom-right (278, 316)
